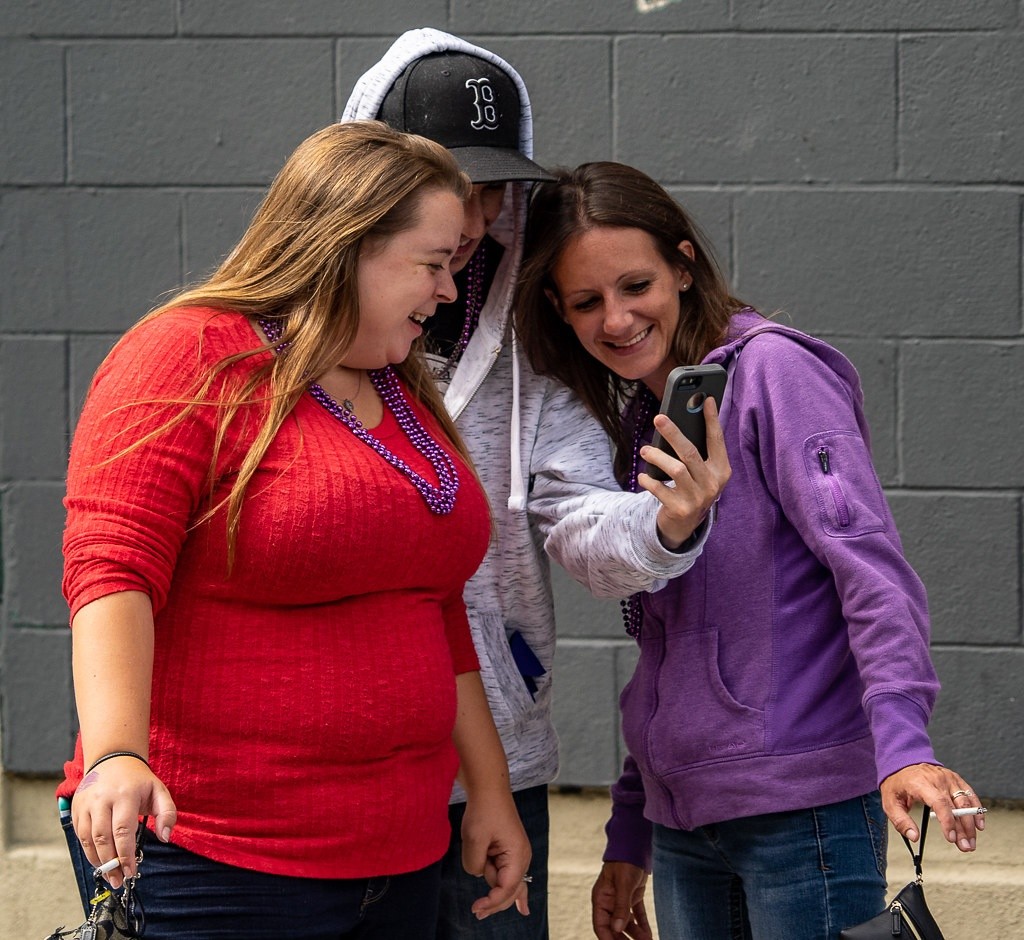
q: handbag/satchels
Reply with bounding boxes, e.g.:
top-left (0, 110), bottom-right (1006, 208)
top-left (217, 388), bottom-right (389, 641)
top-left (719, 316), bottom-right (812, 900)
top-left (839, 805), bottom-right (945, 940)
top-left (47, 755), bottom-right (152, 940)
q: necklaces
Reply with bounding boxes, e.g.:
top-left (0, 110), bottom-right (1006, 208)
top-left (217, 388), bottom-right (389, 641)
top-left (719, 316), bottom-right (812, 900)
top-left (259, 318), bottom-right (458, 514)
top-left (433, 243), bottom-right (487, 377)
top-left (312, 368), bottom-right (361, 412)
top-left (621, 384), bottom-right (649, 638)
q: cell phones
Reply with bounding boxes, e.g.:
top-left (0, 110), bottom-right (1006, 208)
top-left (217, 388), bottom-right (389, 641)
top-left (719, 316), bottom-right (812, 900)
top-left (643, 363), bottom-right (727, 482)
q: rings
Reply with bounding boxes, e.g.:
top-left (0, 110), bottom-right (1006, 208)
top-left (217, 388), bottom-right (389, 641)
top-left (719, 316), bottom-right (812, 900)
top-left (965, 790), bottom-right (975, 796)
top-left (522, 875), bottom-right (532, 882)
top-left (954, 791), bottom-right (965, 800)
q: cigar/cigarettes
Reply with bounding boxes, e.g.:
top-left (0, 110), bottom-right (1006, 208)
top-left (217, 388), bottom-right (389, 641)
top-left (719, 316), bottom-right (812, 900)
top-left (92, 857), bottom-right (123, 876)
top-left (928, 807), bottom-right (990, 817)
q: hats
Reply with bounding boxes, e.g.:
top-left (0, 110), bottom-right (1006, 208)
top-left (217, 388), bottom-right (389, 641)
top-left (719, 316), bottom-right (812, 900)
top-left (375, 52), bottom-right (559, 185)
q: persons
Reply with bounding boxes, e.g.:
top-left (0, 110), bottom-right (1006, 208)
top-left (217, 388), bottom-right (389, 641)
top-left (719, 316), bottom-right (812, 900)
top-left (53, 120), bottom-right (535, 940)
top-left (512, 162), bottom-right (990, 940)
top-left (334, 26), bottom-right (732, 940)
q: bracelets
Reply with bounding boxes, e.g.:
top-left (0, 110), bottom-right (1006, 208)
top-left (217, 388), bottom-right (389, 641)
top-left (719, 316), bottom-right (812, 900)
top-left (84, 751), bottom-right (149, 777)
